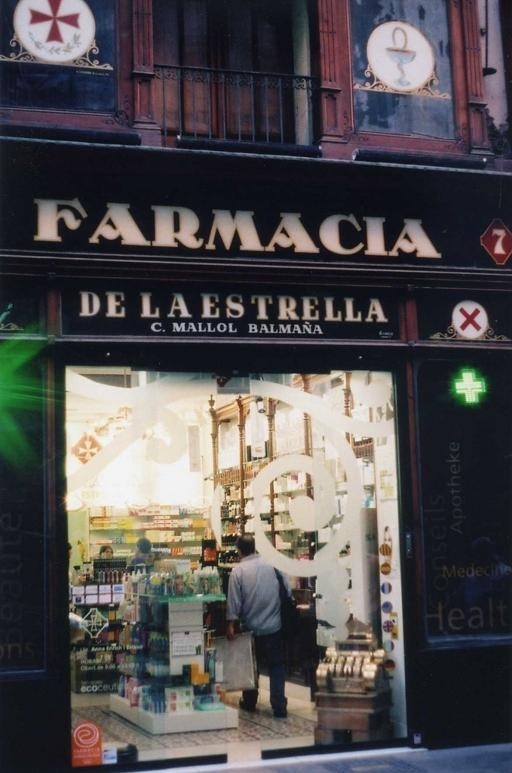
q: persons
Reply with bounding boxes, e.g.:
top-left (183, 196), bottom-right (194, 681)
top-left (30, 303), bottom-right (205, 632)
top-left (125, 538), bottom-right (155, 574)
top-left (226, 534), bottom-right (296, 718)
top-left (99, 545), bottom-right (115, 562)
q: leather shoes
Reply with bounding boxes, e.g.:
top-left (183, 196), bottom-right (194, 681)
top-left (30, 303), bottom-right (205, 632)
top-left (271, 705), bottom-right (288, 717)
top-left (239, 698), bottom-right (256, 711)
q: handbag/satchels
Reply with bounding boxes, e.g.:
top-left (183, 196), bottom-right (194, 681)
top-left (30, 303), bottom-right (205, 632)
top-left (275, 568), bottom-right (302, 636)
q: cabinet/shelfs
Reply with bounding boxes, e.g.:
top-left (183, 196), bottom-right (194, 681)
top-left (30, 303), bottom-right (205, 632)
top-left (207, 467), bottom-right (315, 594)
top-left (86, 494), bottom-right (208, 561)
top-left (111, 568), bottom-right (243, 733)
top-left (64, 568), bottom-right (130, 705)
